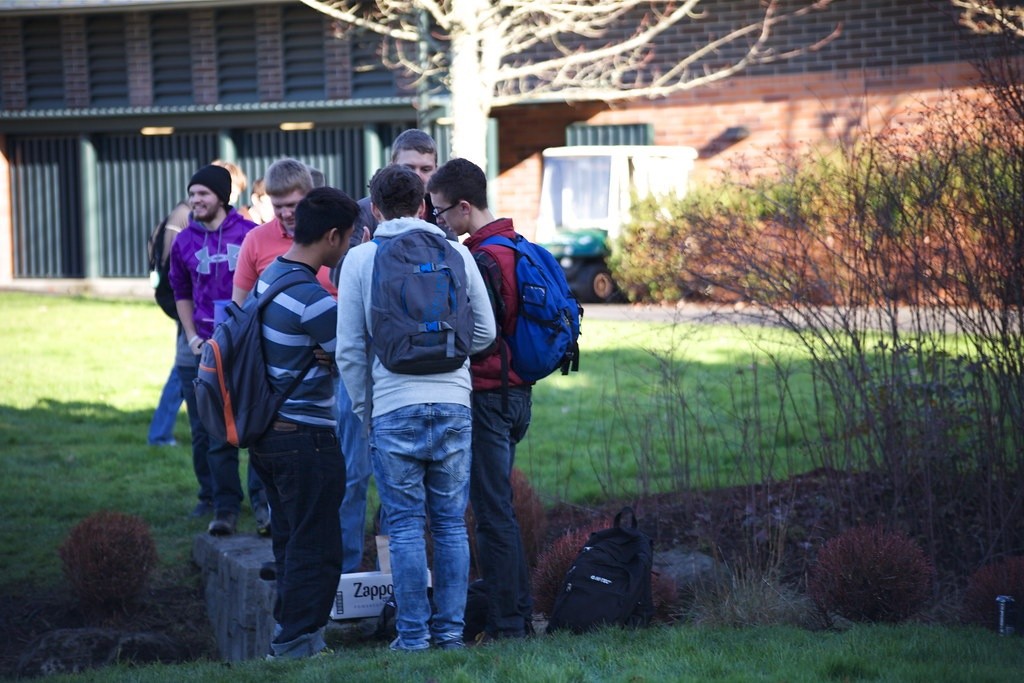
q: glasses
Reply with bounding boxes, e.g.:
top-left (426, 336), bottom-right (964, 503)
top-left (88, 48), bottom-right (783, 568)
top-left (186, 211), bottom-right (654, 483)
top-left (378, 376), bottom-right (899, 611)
top-left (433, 202), bottom-right (459, 217)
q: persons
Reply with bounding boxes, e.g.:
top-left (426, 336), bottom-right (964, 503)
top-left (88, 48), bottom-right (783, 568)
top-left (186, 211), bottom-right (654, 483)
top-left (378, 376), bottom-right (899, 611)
top-left (427, 157), bottom-right (534, 646)
top-left (334, 164), bottom-right (498, 649)
top-left (144, 167), bottom-right (326, 282)
top-left (171, 162), bottom-right (274, 538)
top-left (241, 187), bottom-right (364, 664)
top-left (329, 127), bottom-right (462, 574)
top-left (146, 160), bottom-right (248, 448)
top-left (231, 159), bottom-right (341, 308)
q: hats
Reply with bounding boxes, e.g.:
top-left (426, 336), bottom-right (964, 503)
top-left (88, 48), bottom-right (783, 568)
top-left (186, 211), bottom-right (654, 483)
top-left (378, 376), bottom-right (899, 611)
top-left (189, 166), bottom-right (232, 207)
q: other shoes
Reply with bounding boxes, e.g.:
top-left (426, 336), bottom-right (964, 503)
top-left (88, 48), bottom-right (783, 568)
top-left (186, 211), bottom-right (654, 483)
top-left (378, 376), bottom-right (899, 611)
top-left (390, 634), bottom-right (429, 653)
top-left (266, 648), bottom-right (334, 665)
top-left (440, 640), bottom-right (468, 651)
top-left (149, 437), bottom-right (176, 447)
top-left (474, 632), bottom-right (529, 652)
top-left (209, 509), bottom-right (241, 535)
top-left (192, 499), bottom-right (216, 517)
top-left (258, 520), bottom-right (273, 538)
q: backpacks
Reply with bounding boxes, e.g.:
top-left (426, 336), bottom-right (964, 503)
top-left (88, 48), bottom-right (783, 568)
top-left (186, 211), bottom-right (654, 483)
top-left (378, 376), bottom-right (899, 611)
top-left (550, 508), bottom-right (654, 633)
top-left (482, 234), bottom-right (581, 382)
top-left (193, 270), bottom-right (320, 447)
top-left (373, 228), bottom-right (472, 374)
top-left (148, 202), bottom-right (193, 272)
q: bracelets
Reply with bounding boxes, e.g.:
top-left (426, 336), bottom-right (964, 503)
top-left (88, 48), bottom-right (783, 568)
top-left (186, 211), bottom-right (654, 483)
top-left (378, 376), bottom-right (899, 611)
top-left (187, 334), bottom-right (199, 347)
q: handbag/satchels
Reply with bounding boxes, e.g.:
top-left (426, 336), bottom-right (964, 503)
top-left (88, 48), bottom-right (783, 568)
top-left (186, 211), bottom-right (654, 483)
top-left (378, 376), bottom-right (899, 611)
top-left (375, 580), bottom-right (487, 642)
top-left (156, 250), bottom-right (197, 322)
